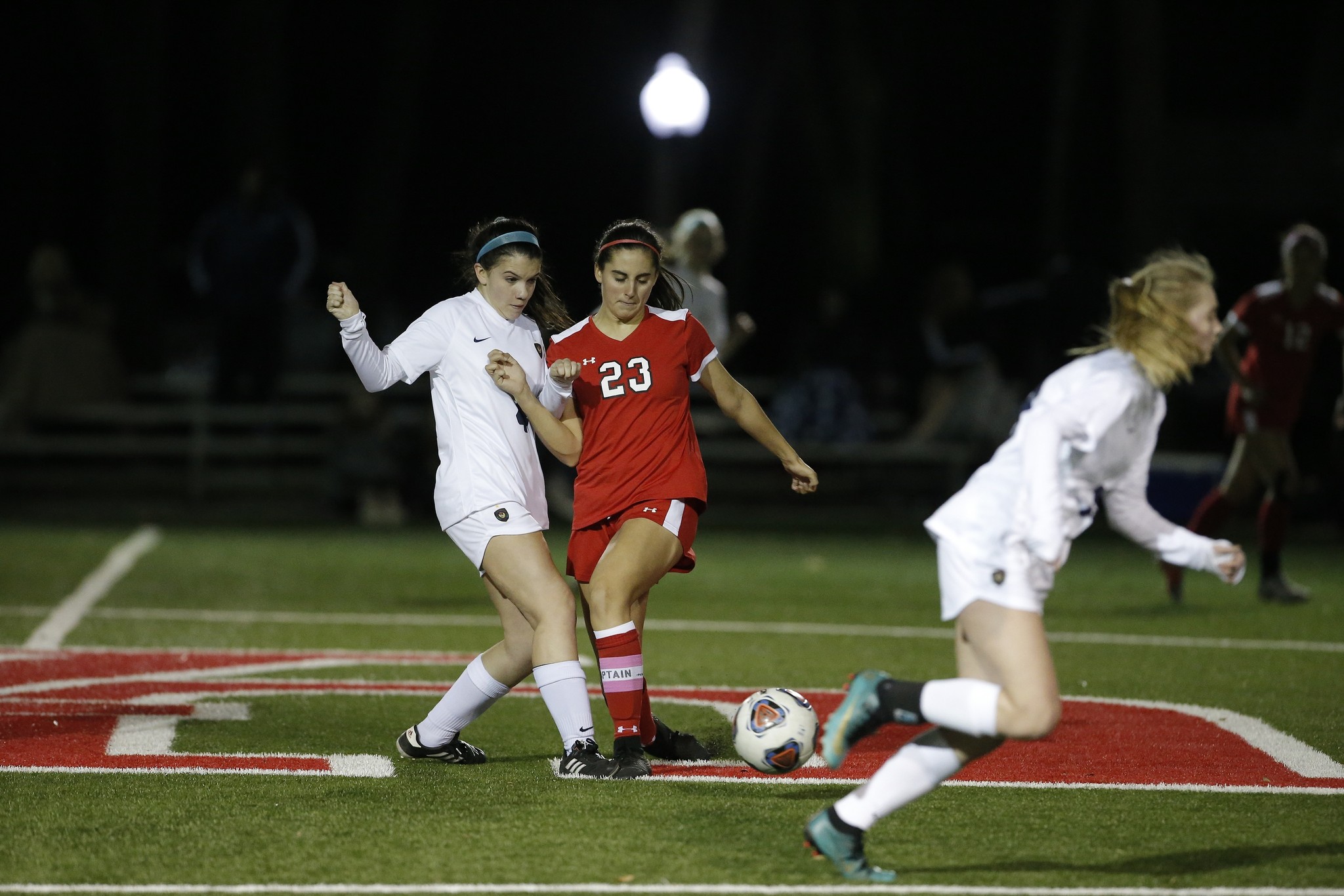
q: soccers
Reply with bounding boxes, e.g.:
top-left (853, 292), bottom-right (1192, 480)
top-left (731, 685), bottom-right (820, 775)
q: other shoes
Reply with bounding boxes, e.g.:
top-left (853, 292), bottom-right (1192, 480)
top-left (1258, 576), bottom-right (1311, 602)
top-left (1156, 551), bottom-right (1186, 601)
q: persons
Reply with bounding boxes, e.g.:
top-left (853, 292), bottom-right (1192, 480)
top-left (12, 235), bottom-right (1011, 456)
top-left (803, 245), bottom-right (1251, 885)
top-left (659, 206), bottom-right (757, 373)
top-left (1156, 218), bottom-right (1344, 613)
top-left (547, 216), bottom-right (819, 778)
top-left (319, 214), bottom-right (619, 781)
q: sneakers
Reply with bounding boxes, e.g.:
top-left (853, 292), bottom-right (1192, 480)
top-left (818, 670), bottom-right (893, 769)
top-left (395, 725), bottom-right (486, 763)
top-left (801, 810), bottom-right (897, 882)
top-left (558, 748), bottom-right (619, 780)
top-left (641, 712), bottom-right (711, 761)
top-left (610, 736), bottom-right (653, 780)
